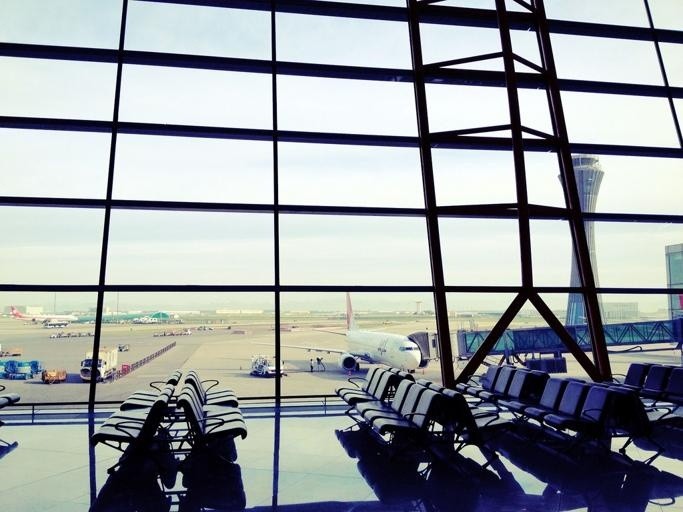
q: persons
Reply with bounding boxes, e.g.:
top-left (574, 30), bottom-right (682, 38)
top-left (310, 359), bottom-right (314, 372)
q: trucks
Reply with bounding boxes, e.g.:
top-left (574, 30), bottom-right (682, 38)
top-left (79, 347), bottom-right (118, 382)
top-left (3, 360), bottom-right (32, 376)
top-left (249, 351), bottom-right (284, 377)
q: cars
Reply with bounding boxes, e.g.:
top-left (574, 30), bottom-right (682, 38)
top-left (46, 330), bottom-right (97, 339)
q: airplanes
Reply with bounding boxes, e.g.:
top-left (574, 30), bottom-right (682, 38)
top-left (251, 293), bottom-right (430, 376)
top-left (10, 303), bottom-right (78, 328)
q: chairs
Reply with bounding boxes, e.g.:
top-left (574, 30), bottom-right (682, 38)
top-left (0, 384), bottom-right (21, 427)
top-left (90, 369), bottom-right (248, 474)
top-left (335, 362), bottom-right (683, 468)
